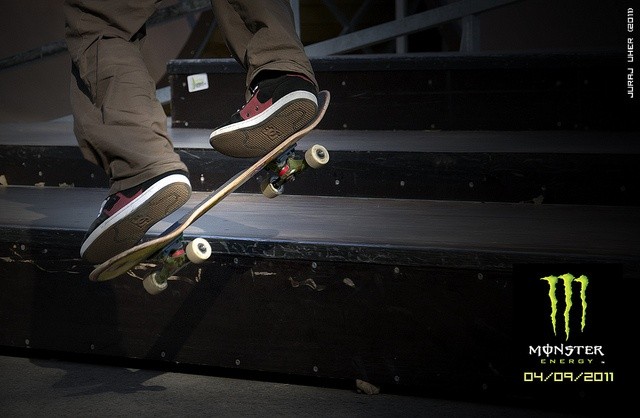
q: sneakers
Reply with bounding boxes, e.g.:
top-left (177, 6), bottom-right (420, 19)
top-left (209, 75), bottom-right (320, 158)
top-left (79, 169), bottom-right (194, 265)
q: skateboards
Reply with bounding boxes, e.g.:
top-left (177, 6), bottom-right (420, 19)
top-left (88, 89), bottom-right (329, 295)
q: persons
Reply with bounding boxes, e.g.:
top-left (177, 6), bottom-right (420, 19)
top-left (62, 0), bottom-right (322, 265)
